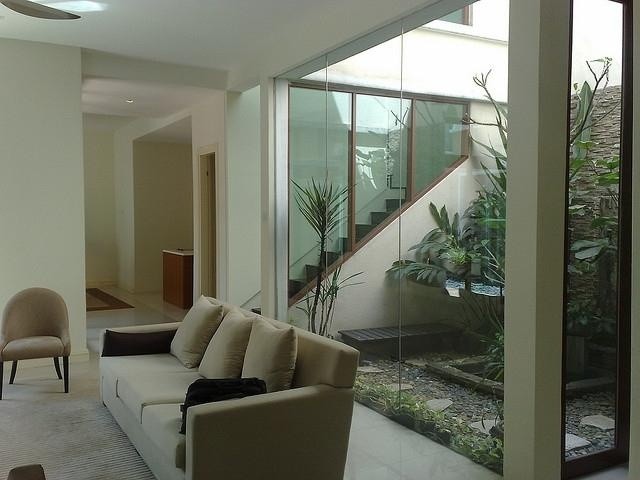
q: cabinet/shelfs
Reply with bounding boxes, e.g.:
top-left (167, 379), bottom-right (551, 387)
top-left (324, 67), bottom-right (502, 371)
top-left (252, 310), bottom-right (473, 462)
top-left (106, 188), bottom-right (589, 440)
top-left (161, 248), bottom-right (194, 310)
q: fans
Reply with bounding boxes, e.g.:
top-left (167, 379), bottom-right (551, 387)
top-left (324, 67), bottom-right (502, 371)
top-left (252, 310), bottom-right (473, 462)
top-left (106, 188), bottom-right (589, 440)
top-left (0, 0), bottom-right (82, 20)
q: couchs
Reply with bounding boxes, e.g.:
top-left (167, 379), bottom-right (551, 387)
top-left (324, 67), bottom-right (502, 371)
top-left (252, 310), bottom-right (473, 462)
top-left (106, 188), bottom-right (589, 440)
top-left (99, 297), bottom-right (361, 480)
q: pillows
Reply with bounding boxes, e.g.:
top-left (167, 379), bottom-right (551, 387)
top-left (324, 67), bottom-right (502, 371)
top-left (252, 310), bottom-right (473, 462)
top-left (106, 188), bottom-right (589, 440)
top-left (100, 294), bottom-right (298, 434)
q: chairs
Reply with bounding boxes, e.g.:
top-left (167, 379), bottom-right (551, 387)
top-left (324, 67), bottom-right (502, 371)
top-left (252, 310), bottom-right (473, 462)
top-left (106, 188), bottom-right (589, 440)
top-left (0, 287), bottom-right (71, 400)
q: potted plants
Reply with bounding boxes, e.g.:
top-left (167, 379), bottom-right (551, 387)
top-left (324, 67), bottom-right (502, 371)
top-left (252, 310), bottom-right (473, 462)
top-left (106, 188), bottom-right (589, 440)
top-left (454, 286), bottom-right (506, 449)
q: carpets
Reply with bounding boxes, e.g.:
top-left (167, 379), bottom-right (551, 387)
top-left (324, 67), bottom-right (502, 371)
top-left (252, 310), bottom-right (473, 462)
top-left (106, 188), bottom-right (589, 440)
top-left (86, 287), bottom-right (135, 311)
